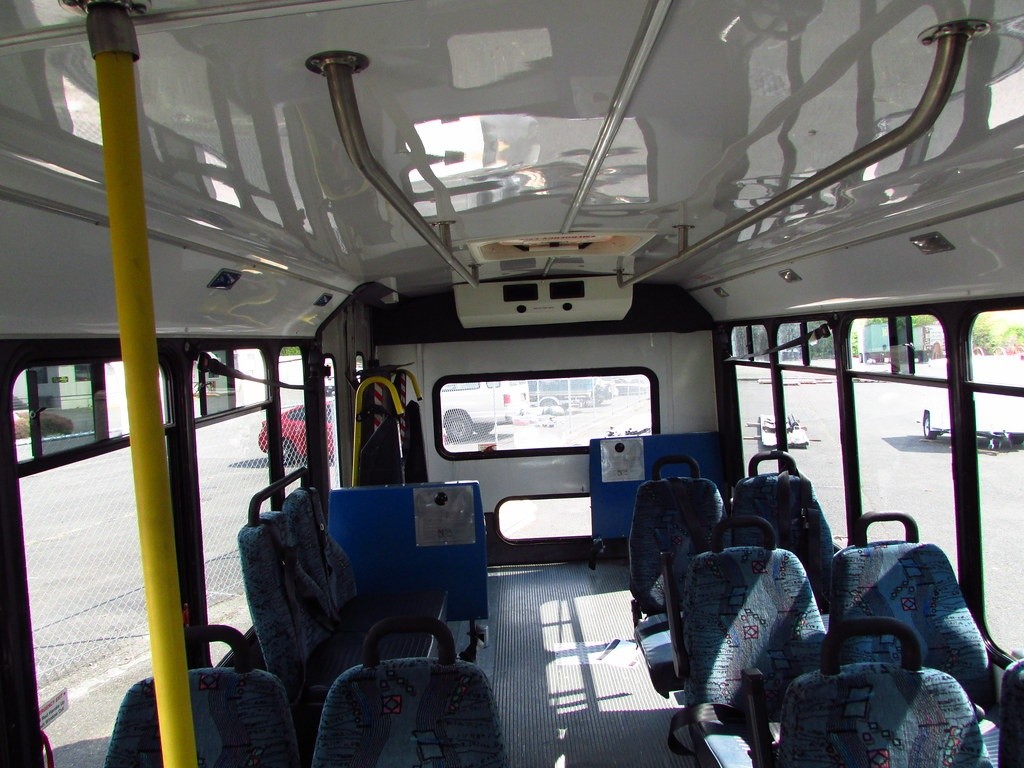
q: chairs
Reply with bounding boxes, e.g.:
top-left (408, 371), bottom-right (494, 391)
top-left (629, 453), bottom-right (1024, 768)
top-left (105, 616), bottom-right (490, 768)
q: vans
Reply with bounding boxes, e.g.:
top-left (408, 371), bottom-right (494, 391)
top-left (443, 379), bottom-right (530, 444)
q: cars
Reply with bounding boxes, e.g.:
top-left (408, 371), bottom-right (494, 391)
top-left (258, 400), bottom-right (336, 466)
top-left (917, 355), bottom-right (1024, 447)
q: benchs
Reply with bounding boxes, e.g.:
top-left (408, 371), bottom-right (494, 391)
top-left (240, 467), bottom-right (448, 709)
